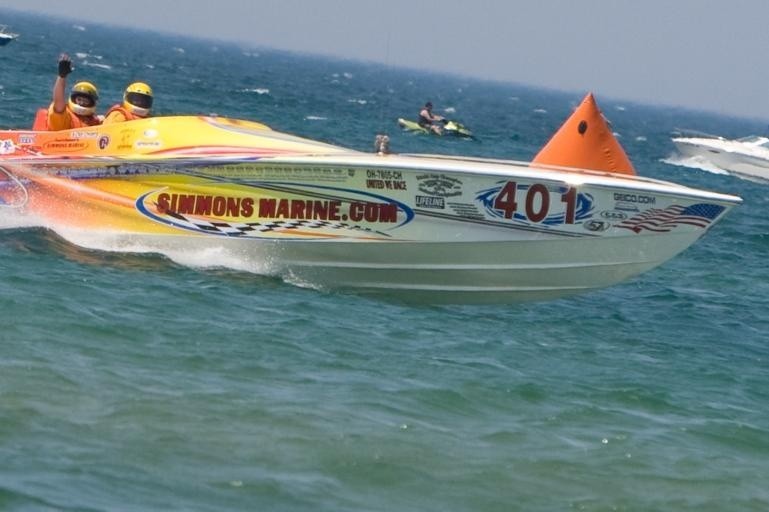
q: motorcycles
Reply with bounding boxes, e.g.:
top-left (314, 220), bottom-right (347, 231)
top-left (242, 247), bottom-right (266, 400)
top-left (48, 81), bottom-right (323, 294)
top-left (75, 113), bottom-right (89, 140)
top-left (395, 115), bottom-right (480, 143)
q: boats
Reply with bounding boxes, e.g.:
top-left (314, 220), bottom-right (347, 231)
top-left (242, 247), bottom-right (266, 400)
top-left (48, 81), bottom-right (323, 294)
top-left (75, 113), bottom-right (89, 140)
top-left (669, 135), bottom-right (768, 185)
top-left (0, 20), bottom-right (23, 50)
top-left (0, 84), bottom-right (746, 308)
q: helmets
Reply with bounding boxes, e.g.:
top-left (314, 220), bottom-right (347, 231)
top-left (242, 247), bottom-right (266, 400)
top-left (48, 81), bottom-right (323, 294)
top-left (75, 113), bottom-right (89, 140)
top-left (122, 82), bottom-right (154, 117)
top-left (67, 82), bottom-right (98, 117)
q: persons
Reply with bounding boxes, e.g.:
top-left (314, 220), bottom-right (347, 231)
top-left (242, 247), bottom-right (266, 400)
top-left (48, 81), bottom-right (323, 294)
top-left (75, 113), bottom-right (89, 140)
top-left (47, 52), bottom-right (103, 133)
top-left (102, 82), bottom-right (152, 123)
top-left (418, 102), bottom-right (445, 137)
